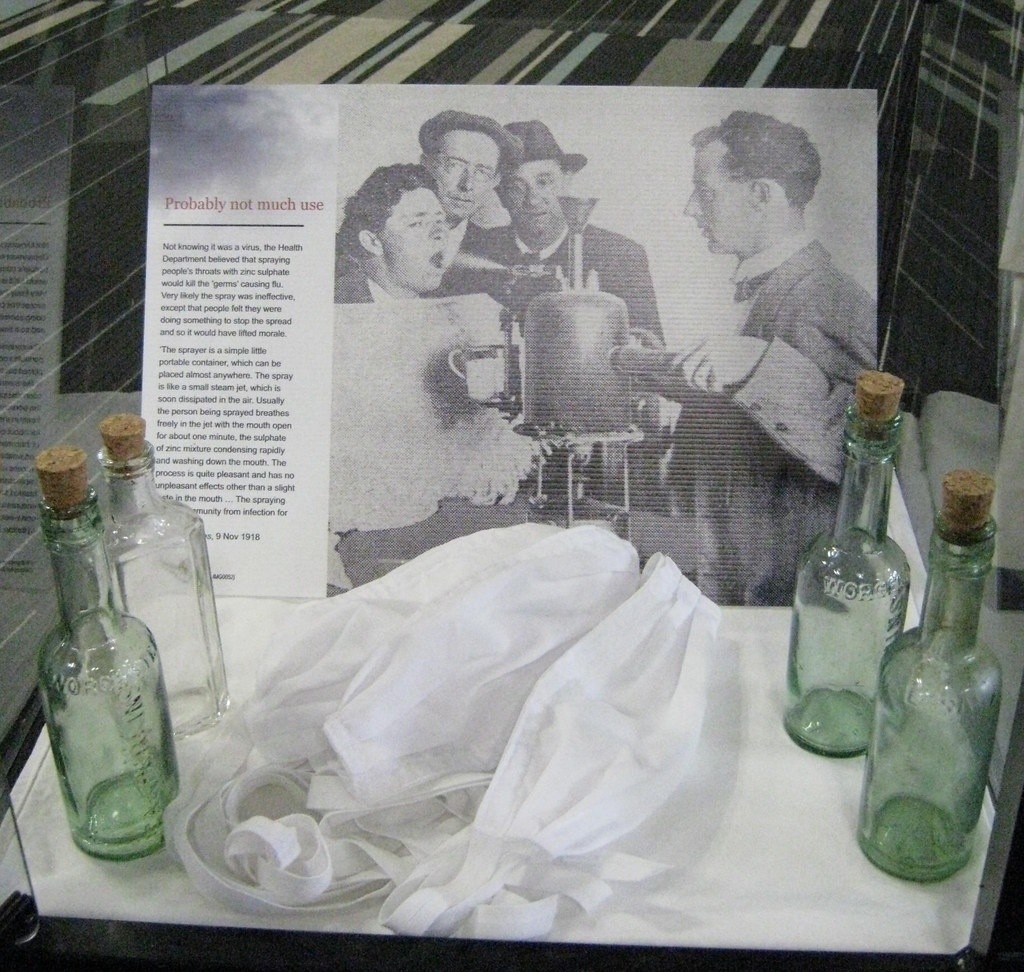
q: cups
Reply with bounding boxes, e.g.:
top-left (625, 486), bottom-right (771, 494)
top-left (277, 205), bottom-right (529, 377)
top-left (448, 347), bottom-right (505, 403)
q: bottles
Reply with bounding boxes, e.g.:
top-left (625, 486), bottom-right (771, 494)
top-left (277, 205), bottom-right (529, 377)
top-left (784, 370), bottom-right (911, 757)
top-left (0, 752), bottom-right (39, 947)
top-left (99, 412), bottom-right (228, 740)
top-left (33, 448), bottom-right (179, 862)
top-left (858, 469), bottom-right (1002, 883)
